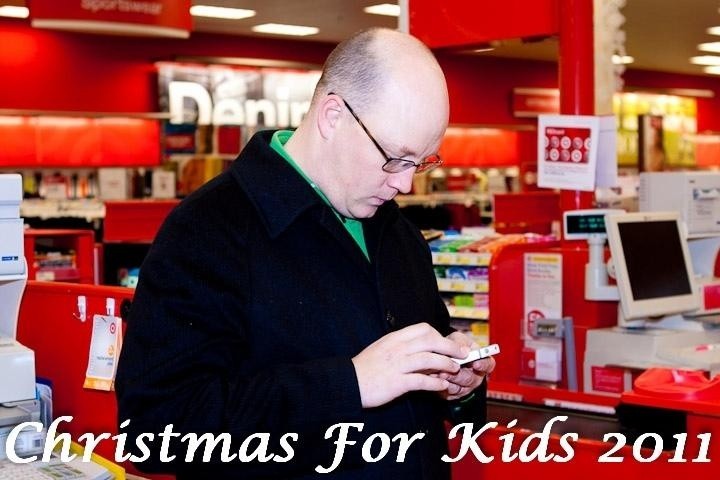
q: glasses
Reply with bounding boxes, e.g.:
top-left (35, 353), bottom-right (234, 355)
top-left (328, 92), bottom-right (443, 174)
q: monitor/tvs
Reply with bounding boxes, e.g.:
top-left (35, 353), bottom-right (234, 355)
top-left (604, 211), bottom-right (701, 329)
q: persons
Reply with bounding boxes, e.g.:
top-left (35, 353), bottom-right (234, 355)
top-left (112, 27), bottom-right (495, 479)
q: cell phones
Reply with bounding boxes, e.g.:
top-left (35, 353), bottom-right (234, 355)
top-left (451, 344), bottom-right (500, 366)
